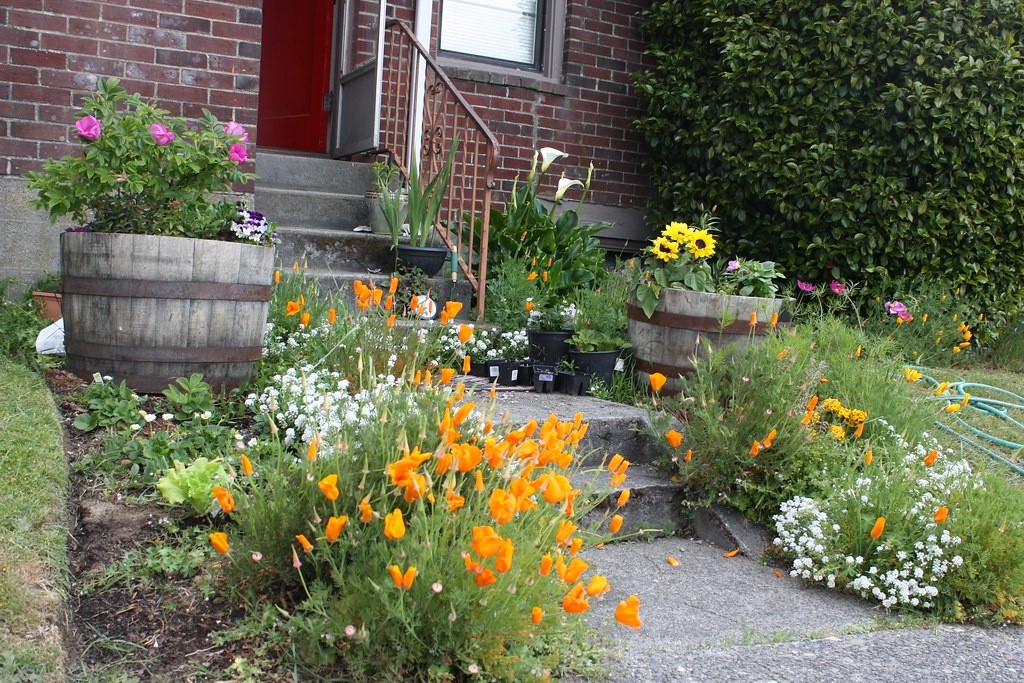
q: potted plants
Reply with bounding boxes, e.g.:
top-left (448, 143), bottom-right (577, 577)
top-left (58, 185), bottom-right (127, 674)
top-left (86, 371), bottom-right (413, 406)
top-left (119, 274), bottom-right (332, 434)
top-left (564, 329), bottom-right (633, 393)
top-left (374, 131), bottom-right (462, 278)
top-left (32, 269), bottom-right (63, 322)
top-left (366, 162), bottom-right (410, 236)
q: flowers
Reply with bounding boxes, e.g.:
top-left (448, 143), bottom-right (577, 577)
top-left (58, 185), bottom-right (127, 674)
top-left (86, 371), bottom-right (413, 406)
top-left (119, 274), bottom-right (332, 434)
top-left (630, 221), bottom-right (787, 319)
top-left (25, 75), bottom-right (278, 248)
top-left (525, 288), bottom-right (583, 332)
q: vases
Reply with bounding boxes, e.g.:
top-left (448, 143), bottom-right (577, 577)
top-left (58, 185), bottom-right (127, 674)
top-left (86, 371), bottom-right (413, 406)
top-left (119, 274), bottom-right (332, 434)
top-left (627, 282), bottom-right (782, 402)
top-left (531, 365), bottom-right (558, 393)
top-left (518, 360), bottom-right (535, 387)
top-left (61, 230), bottom-right (274, 398)
top-left (486, 358), bottom-right (507, 383)
top-left (557, 369), bottom-right (591, 395)
top-left (470, 362), bottom-right (487, 377)
top-left (527, 325), bottom-right (576, 366)
top-left (500, 361), bottom-right (520, 386)
